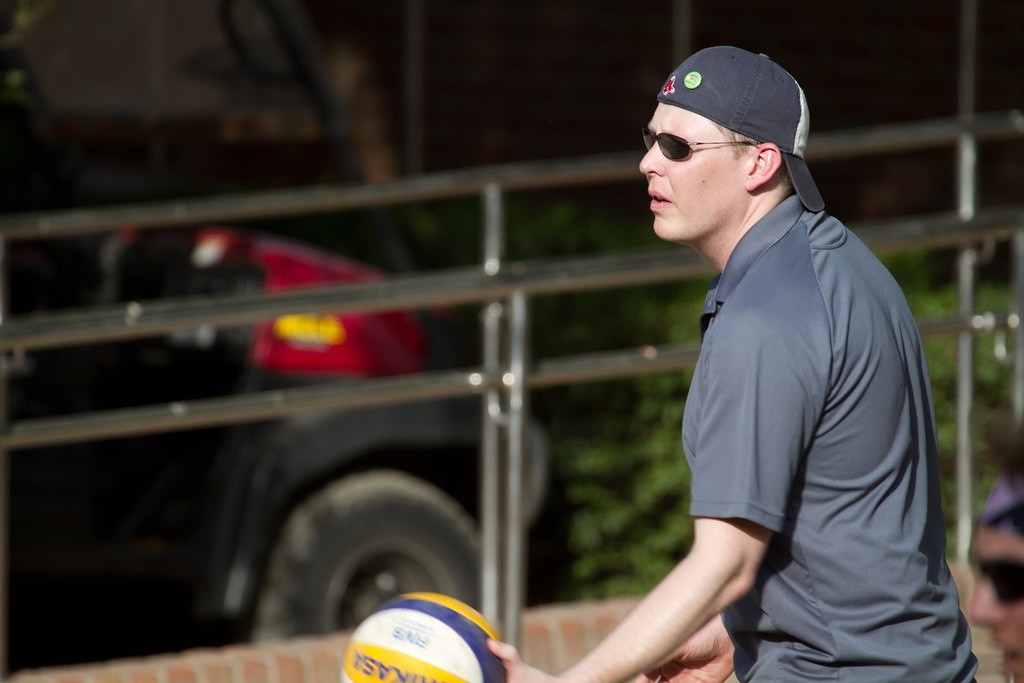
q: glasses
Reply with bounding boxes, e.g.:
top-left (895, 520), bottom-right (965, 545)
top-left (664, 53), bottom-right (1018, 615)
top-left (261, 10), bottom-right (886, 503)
top-left (640, 127), bottom-right (757, 162)
top-left (969, 556), bottom-right (1024, 602)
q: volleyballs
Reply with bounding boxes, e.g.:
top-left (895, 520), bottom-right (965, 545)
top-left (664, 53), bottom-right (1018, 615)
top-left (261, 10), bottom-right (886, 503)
top-left (338, 590), bottom-right (509, 683)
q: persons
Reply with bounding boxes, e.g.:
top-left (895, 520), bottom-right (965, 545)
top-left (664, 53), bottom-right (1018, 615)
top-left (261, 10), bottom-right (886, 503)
top-left (963, 417), bottom-right (1024, 683)
top-left (489, 45), bottom-right (979, 683)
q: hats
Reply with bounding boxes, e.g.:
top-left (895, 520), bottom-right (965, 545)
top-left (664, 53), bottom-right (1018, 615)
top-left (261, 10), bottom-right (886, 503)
top-left (658, 46), bottom-right (827, 213)
